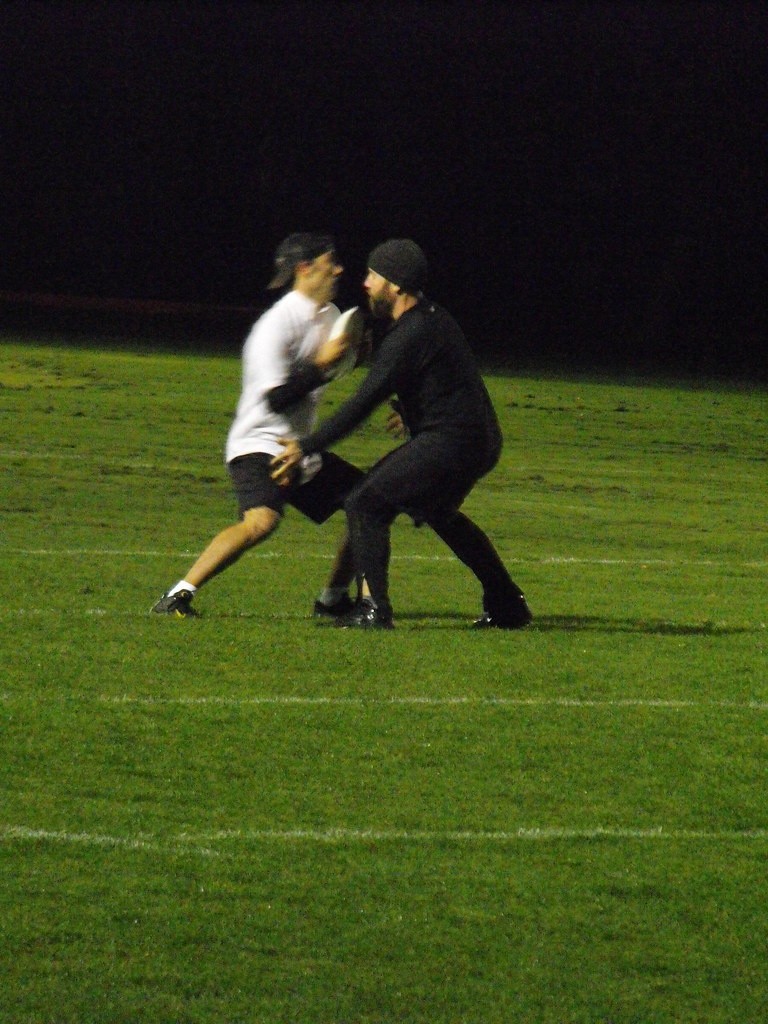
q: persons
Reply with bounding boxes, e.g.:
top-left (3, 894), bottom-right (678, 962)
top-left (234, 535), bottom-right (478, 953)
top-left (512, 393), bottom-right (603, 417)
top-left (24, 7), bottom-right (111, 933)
top-left (269, 239), bottom-right (532, 631)
top-left (151, 232), bottom-right (372, 620)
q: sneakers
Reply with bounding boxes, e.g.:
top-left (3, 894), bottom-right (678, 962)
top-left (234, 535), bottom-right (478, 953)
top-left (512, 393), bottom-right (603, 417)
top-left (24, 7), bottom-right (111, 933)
top-left (313, 591), bottom-right (357, 620)
top-left (150, 590), bottom-right (201, 619)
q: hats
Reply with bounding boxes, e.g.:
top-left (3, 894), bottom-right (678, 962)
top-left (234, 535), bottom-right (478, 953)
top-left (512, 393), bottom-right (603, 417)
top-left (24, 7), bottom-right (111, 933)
top-left (366, 237), bottom-right (431, 294)
top-left (274, 232), bottom-right (341, 277)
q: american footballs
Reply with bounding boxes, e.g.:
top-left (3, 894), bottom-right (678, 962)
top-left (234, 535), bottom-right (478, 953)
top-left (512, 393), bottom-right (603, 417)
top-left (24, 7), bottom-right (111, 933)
top-left (322, 305), bottom-right (365, 384)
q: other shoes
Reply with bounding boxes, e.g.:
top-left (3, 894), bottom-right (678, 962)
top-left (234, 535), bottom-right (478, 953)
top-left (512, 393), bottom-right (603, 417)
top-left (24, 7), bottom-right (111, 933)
top-left (339, 604), bottom-right (395, 629)
top-left (471, 594), bottom-right (533, 631)
